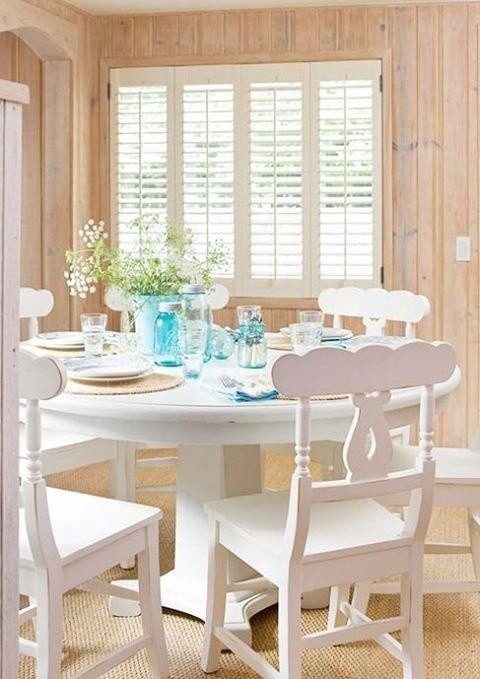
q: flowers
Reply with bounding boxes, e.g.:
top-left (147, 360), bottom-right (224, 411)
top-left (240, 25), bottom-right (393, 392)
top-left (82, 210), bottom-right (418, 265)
top-left (62, 213), bottom-right (233, 328)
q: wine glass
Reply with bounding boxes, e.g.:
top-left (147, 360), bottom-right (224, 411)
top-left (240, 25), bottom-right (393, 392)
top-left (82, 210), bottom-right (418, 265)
top-left (237, 305), bottom-right (262, 323)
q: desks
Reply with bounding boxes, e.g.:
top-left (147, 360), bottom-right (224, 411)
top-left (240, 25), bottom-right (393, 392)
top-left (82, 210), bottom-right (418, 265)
top-left (19, 330), bottom-right (462, 653)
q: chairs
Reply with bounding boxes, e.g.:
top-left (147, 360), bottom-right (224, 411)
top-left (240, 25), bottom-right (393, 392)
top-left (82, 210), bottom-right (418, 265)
top-left (18, 346), bottom-right (175, 679)
top-left (199, 337), bottom-right (460, 679)
top-left (19, 288), bottom-right (137, 572)
top-left (103, 287), bottom-right (232, 502)
top-left (259, 285), bottom-right (429, 494)
top-left (347, 423), bottom-right (479, 636)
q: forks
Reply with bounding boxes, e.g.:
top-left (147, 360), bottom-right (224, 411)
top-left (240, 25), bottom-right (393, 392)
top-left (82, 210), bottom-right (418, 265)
top-left (220, 374), bottom-right (245, 391)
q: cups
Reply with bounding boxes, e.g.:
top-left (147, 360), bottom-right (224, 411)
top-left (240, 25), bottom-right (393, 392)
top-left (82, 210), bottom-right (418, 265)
top-left (79, 313), bottom-right (108, 355)
top-left (289, 310), bottom-right (324, 354)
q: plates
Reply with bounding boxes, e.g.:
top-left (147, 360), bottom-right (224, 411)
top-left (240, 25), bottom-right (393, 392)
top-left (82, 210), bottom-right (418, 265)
top-left (30, 331), bottom-right (84, 349)
top-left (61, 356), bottom-right (154, 380)
top-left (281, 327), bottom-right (354, 342)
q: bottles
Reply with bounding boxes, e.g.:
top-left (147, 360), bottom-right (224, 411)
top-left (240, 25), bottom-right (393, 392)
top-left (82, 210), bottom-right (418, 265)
top-left (154, 285), bottom-right (266, 368)
